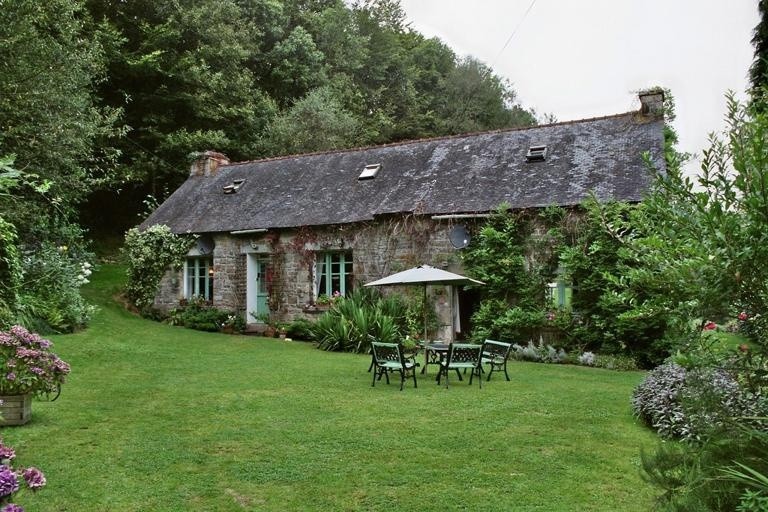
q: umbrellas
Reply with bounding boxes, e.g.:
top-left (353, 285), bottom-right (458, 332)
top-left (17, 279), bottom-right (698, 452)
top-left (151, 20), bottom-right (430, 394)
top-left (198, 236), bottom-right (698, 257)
top-left (359, 261), bottom-right (490, 358)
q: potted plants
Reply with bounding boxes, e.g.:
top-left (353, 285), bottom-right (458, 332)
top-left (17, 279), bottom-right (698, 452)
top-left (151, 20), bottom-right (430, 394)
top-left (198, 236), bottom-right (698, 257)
top-left (218, 313), bottom-right (296, 339)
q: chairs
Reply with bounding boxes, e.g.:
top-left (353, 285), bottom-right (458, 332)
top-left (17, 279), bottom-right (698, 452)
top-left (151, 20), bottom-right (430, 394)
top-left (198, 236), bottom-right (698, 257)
top-left (437, 342), bottom-right (483, 390)
top-left (370, 341), bottom-right (419, 390)
top-left (463, 339), bottom-right (514, 382)
top-left (367, 353), bottom-right (418, 379)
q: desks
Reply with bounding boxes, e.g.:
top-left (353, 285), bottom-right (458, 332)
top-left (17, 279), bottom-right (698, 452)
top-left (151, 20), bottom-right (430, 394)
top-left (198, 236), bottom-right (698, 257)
top-left (420, 342), bottom-right (464, 383)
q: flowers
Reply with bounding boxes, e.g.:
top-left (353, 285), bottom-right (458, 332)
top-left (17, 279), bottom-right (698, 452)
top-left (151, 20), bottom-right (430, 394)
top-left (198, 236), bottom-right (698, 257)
top-left (0, 440), bottom-right (48, 512)
top-left (0, 317), bottom-right (69, 395)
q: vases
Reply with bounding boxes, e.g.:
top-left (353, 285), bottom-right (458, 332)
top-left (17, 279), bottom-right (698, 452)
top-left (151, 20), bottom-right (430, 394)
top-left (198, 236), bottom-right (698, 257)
top-left (0, 386), bottom-right (32, 427)
